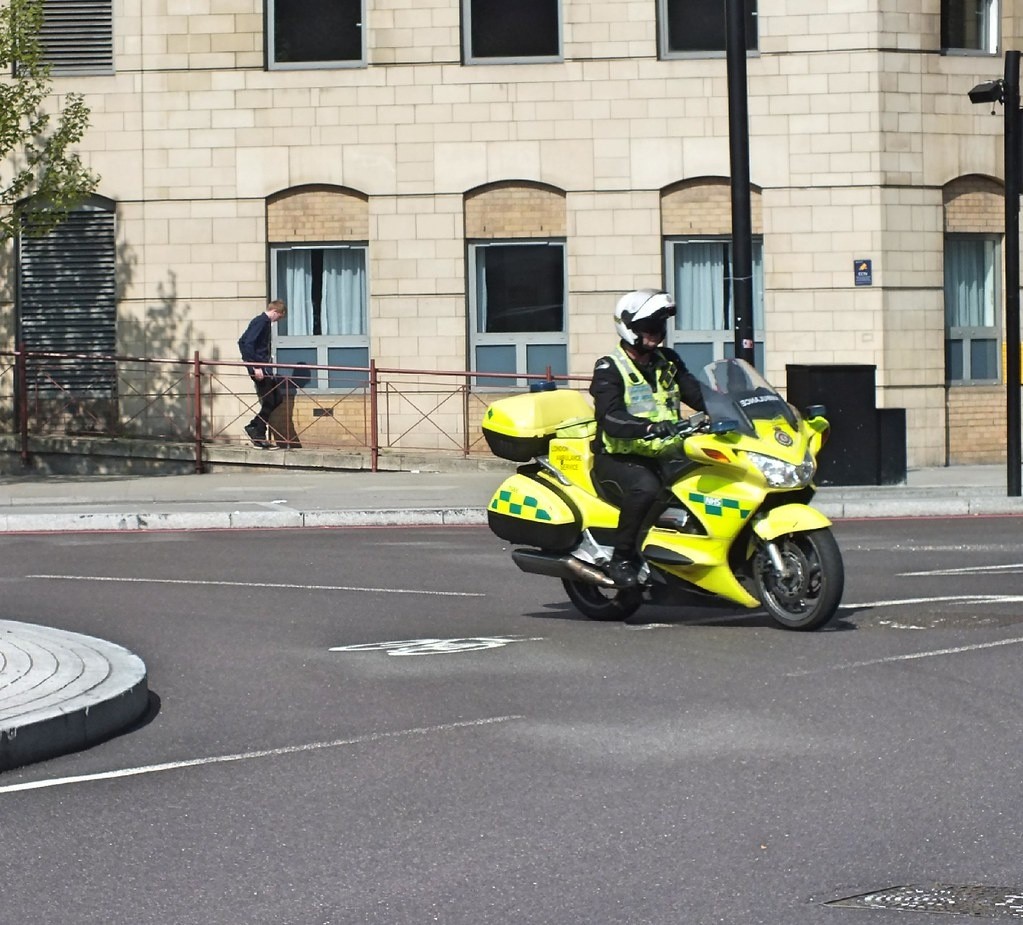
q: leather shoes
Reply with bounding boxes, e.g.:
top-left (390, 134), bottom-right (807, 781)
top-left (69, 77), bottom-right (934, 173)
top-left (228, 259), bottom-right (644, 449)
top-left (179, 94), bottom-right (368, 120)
top-left (608, 560), bottom-right (636, 587)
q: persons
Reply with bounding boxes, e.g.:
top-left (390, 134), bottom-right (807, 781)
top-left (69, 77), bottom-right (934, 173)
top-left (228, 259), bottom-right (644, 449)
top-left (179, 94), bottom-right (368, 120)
top-left (590, 289), bottom-right (734, 591)
top-left (238, 301), bottom-right (287, 450)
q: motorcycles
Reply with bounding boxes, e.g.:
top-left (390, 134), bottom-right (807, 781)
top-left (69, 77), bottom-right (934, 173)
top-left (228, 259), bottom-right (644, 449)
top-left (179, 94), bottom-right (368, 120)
top-left (482, 353), bottom-right (846, 631)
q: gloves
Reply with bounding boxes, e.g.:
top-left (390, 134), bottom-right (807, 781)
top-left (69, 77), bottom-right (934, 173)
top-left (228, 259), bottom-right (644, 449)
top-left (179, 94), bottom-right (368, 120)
top-left (650, 420), bottom-right (678, 439)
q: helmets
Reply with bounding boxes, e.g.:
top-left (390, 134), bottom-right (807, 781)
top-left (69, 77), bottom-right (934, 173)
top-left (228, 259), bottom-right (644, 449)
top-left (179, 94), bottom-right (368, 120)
top-left (614, 288), bottom-right (677, 349)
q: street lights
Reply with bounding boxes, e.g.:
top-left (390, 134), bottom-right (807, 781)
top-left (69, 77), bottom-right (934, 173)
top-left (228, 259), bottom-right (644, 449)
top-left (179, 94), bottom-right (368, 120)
top-left (970, 79), bottom-right (1020, 500)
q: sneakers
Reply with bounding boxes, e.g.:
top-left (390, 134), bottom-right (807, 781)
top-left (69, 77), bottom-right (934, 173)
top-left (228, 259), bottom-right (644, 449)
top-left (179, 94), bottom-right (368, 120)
top-left (242, 425), bottom-right (280, 450)
